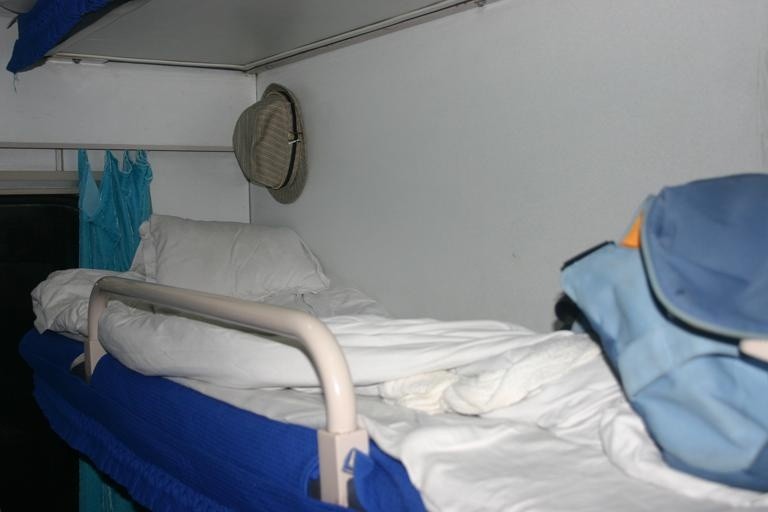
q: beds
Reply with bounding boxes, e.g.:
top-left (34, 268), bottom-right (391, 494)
top-left (17, 256), bottom-right (767, 512)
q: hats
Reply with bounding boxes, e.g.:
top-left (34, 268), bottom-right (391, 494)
top-left (231, 82), bottom-right (310, 205)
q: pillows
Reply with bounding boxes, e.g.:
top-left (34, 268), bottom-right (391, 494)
top-left (138, 211), bottom-right (332, 310)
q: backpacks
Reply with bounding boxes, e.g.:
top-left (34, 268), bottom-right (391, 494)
top-left (557, 169), bottom-right (768, 496)
